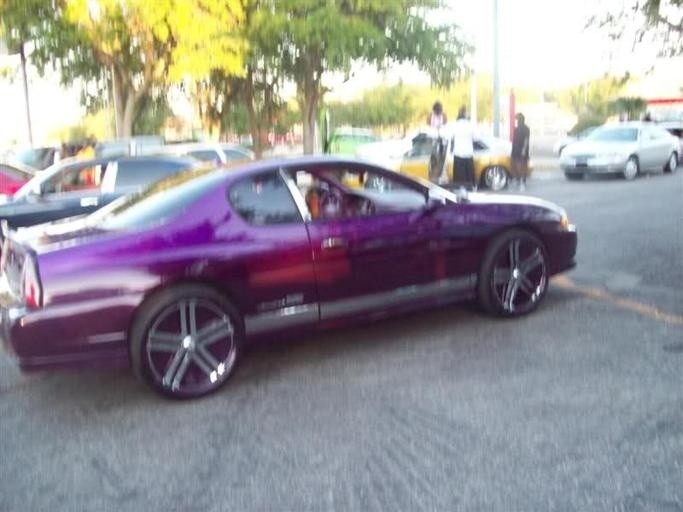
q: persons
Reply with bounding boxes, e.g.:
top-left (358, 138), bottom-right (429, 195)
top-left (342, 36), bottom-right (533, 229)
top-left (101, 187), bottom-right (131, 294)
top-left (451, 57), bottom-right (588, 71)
top-left (509, 113), bottom-right (531, 195)
top-left (70, 137), bottom-right (97, 185)
top-left (426, 100), bottom-right (448, 130)
top-left (442, 106), bottom-right (478, 193)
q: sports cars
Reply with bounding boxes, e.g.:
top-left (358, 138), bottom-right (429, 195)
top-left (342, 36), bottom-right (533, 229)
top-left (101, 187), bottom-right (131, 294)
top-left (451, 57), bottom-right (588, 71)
top-left (0, 154), bottom-right (578, 401)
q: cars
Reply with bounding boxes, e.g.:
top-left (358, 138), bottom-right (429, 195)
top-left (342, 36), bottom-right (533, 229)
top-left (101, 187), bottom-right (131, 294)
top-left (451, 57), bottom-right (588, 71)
top-left (1, 137), bottom-right (259, 233)
top-left (342, 124), bottom-right (535, 192)
top-left (555, 119), bottom-right (683, 179)
